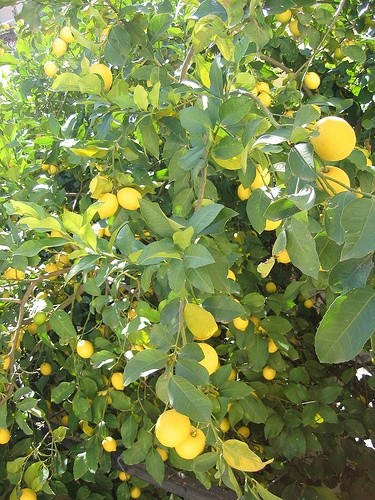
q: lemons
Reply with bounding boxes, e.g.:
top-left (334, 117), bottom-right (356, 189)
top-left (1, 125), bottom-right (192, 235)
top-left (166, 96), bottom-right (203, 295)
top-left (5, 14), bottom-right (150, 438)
top-left (0, 0), bottom-right (375, 500)
